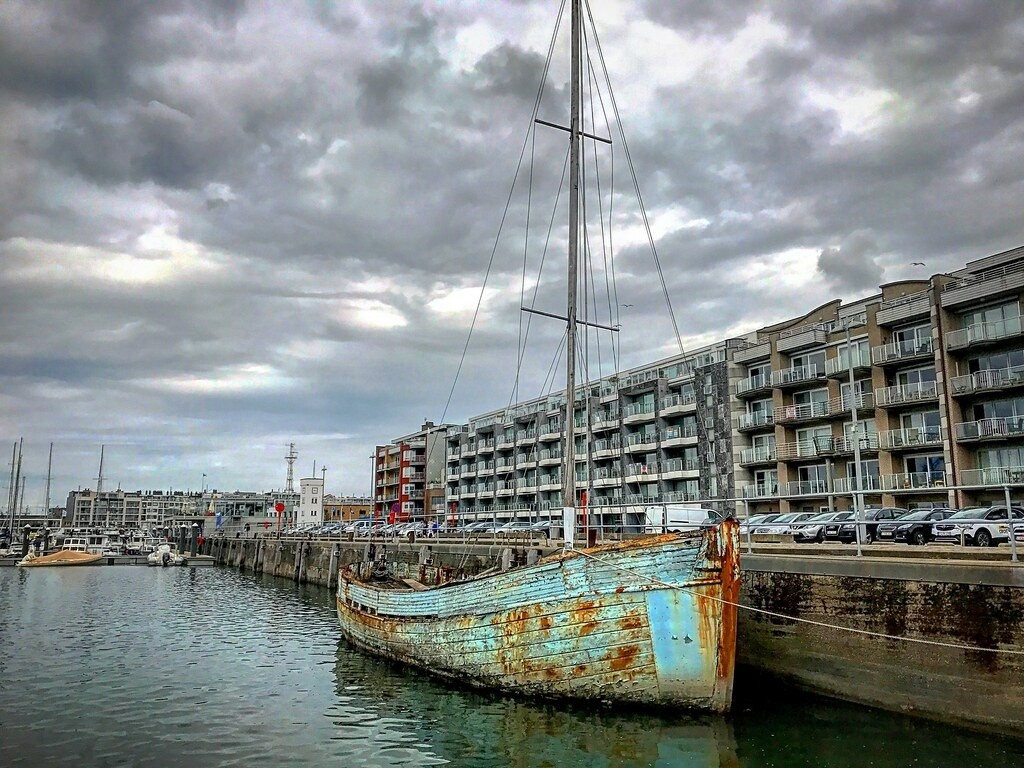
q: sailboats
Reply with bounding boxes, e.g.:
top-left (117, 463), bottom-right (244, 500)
top-left (334, 9), bottom-right (743, 717)
top-left (14, 548), bottom-right (104, 567)
top-left (0, 437), bottom-right (168, 559)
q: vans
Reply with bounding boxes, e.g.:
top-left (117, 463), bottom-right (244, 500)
top-left (644, 505), bottom-right (724, 534)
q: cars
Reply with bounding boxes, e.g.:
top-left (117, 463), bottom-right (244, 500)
top-left (469, 522), bottom-right (506, 533)
top-left (262, 520), bottom-right (465, 538)
top-left (484, 521), bottom-right (535, 533)
top-left (821, 507), bottom-right (910, 544)
top-left (739, 512), bottom-right (784, 535)
top-left (791, 510), bottom-right (858, 544)
top-left (931, 505), bottom-right (1024, 547)
top-left (524, 520), bottom-right (565, 539)
top-left (876, 507), bottom-right (960, 545)
top-left (754, 511), bottom-right (820, 543)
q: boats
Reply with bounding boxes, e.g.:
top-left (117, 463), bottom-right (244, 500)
top-left (147, 544), bottom-right (184, 566)
